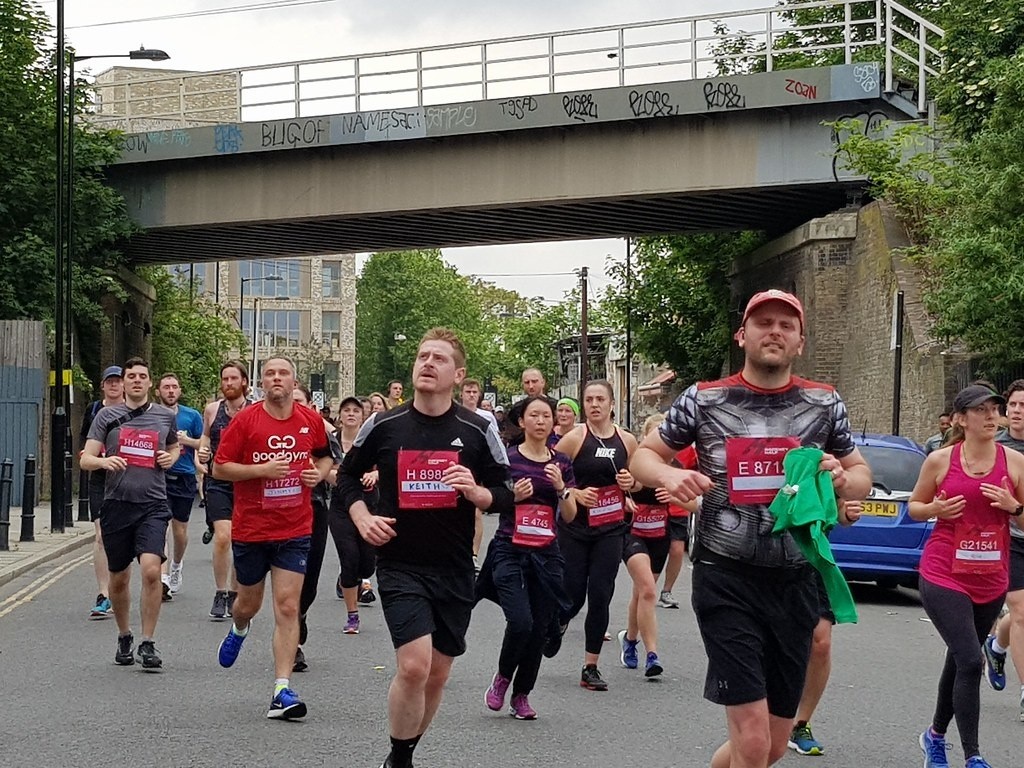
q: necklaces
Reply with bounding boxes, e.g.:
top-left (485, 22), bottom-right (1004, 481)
top-left (962, 444), bottom-right (993, 475)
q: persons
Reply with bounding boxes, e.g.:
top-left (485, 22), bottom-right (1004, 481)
top-left (335, 328), bottom-right (516, 768)
top-left (920, 380), bottom-right (1024, 724)
top-left (484, 394), bottom-right (579, 720)
top-left (210, 352), bottom-right (337, 721)
top-left (908, 384), bottom-right (1024, 768)
top-left (628, 289), bottom-right (872, 768)
top-left (554, 380), bottom-right (644, 693)
top-left (77, 354), bottom-right (703, 673)
top-left (785, 491), bottom-right (862, 756)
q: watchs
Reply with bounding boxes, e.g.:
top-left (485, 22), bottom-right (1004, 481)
top-left (556, 487), bottom-right (571, 502)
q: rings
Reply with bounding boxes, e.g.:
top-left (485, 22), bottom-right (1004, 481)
top-left (831, 470), bottom-right (838, 479)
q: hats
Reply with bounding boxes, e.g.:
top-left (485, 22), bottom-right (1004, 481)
top-left (102, 366), bottom-right (122, 382)
top-left (954, 385), bottom-right (1005, 414)
top-left (340, 397), bottom-right (364, 409)
top-left (495, 406), bottom-right (504, 413)
top-left (741, 289), bottom-right (804, 329)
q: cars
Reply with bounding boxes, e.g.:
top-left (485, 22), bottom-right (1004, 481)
top-left (823, 430), bottom-right (941, 596)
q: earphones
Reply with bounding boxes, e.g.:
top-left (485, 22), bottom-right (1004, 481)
top-left (610, 405), bottom-right (613, 412)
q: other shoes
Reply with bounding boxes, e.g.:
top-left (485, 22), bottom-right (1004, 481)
top-left (201, 526), bottom-right (214, 545)
top-left (604, 632), bottom-right (611, 640)
top-left (292, 648), bottom-right (308, 672)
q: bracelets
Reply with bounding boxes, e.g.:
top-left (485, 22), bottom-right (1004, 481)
top-left (628, 479), bottom-right (637, 492)
top-left (1008, 504), bottom-right (1024, 516)
top-left (844, 511), bottom-right (860, 524)
top-left (555, 485), bottom-right (566, 493)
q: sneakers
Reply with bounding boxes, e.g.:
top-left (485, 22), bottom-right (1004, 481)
top-left (136, 641), bottom-right (163, 672)
top-left (209, 591), bottom-right (238, 622)
top-left (981, 634), bottom-right (1006, 691)
top-left (89, 593), bottom-right (114, 619)
top-left (169, 561), bottom-right (184, 592)
top-left (357, 582), bottom-right (376, 603)
top-left (657, 591), bottom-right (680, 608)
top-left (266, 688), bottom-right (307, 719)
top-left (1019, 686), bottom-right (1024, 722)
top-left (510, 692), bottom-right (538, 720)
top-left (787, 720), bottom-right (825, 755)
top-left (543, 617), bottom-right (568, 659)
top-left (336, 573), bottom-right (344, 598)
top-left (919, 723), bottom-right (953, 768)
top-left (645, 651), bottom-right (664, 677)
top-left (343, 615), bottom-right (360, 634)
top-left (964, 756), bottom-right (994, 768)
top-left (581, 664), bottom-right (609, 691)
top-left (114, 632), bottom-right (135, 665)
top-left (218, 624), bottom-right (248, 668)
top-left (161, 574), bottom-right (172, 601)
top-left (484, 672), bottom-right (510, 712)
top-left (618, 630), bottom-right (641, 669)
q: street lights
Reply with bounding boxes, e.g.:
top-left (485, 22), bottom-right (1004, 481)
top-left (54, 49), bottom-right (172, 527)
top-left (238, 274), bottom-right (283, 359)
top-left (251, 295), bottom-right (291, 386)
